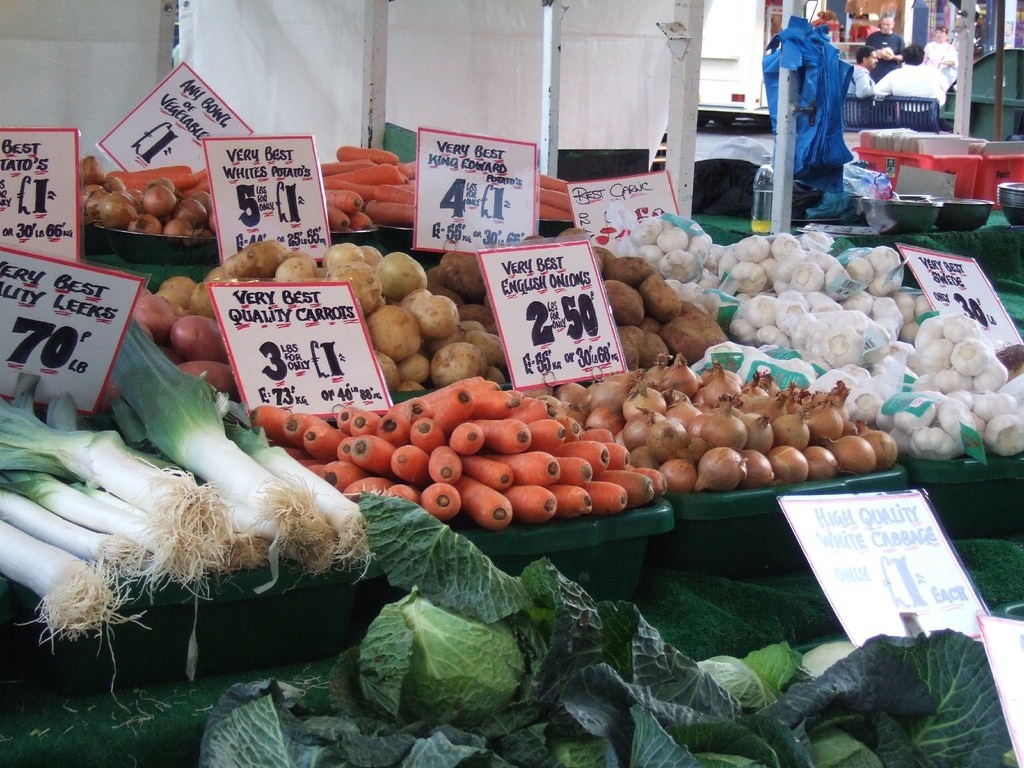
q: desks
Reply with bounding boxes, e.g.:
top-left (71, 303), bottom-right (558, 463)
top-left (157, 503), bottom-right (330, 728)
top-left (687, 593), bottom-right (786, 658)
top-left (691, 206), bottom-right (1024, 292)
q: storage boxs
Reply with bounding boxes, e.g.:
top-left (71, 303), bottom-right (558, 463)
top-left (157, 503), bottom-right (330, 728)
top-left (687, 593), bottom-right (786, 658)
top-left (9, 454), bottom-right (1024, 693)
top-left (982, 154), bottom-right (1024, 210)
top-left (853, 147), bottom-right (983, 198)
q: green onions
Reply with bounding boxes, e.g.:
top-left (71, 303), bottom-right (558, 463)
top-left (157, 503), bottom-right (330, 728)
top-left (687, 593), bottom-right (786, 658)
top-left (0, 319), bottom-right (369, 643)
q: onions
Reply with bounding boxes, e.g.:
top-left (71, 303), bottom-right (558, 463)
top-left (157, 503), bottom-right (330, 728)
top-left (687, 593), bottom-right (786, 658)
top-left (525, 352), bottom-right (898, 492)
top-left (81, 156), bottom-right (219, 237)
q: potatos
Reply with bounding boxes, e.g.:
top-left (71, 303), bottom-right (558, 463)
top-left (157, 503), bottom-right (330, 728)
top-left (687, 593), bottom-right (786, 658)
top-left (129, 226), bottom-right (729, 419)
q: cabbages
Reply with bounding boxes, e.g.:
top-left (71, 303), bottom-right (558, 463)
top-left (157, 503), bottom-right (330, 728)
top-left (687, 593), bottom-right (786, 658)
top-left (197, 498), bottom-right (1014, 768)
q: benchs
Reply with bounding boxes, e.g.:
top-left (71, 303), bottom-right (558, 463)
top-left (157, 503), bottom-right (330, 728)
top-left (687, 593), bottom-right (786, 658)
top-left (842, 93), bottom-right (953, 134)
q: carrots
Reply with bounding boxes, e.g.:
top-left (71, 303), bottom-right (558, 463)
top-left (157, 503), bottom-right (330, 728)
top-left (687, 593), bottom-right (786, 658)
top-left (107, 144), bottom-right (417, 231)
top-left (248, 375), bottom-right (667, 530)
top-left (539, 174), bottom-right (572, 220)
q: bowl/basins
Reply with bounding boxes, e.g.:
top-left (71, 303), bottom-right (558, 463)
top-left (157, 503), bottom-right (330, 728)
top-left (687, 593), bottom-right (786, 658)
top-left (372, 222), bottom-right (414, 252)
top-left (538, 218), bottom-right (573, 237)
top-left (326, 226), bottom-right (378, 249)
top-left (925, 197), bottom-right (996, 232)
top-left (78, 219), bottom-right (111, 254)
top-left (846, 194), bottom-right (930, 216)
top-left (95, 223), bottom-right (219, 264)
top-left (996, 183), bottom-right (1024, 226)
top-left (861, 202), bottom-right (943, 236)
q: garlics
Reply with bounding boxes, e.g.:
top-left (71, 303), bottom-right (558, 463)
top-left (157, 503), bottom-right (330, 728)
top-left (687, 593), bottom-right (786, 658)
top-left (620, 216), bottom-right (1024, 461)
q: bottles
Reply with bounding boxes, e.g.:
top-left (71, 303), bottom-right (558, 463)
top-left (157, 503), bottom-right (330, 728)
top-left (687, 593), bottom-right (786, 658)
top-left (752, 155), bottom-right (774, 233)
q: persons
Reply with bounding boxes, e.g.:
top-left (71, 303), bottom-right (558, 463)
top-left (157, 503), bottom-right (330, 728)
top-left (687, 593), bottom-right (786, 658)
top-left (875, 45), bottom-right (950, 134)
top-left (865, 13), bottom-right (906, 85)
top-left (922, 25), bottom-right (959, 93)
top-left (842, 46), bottom-right (878, 125)
